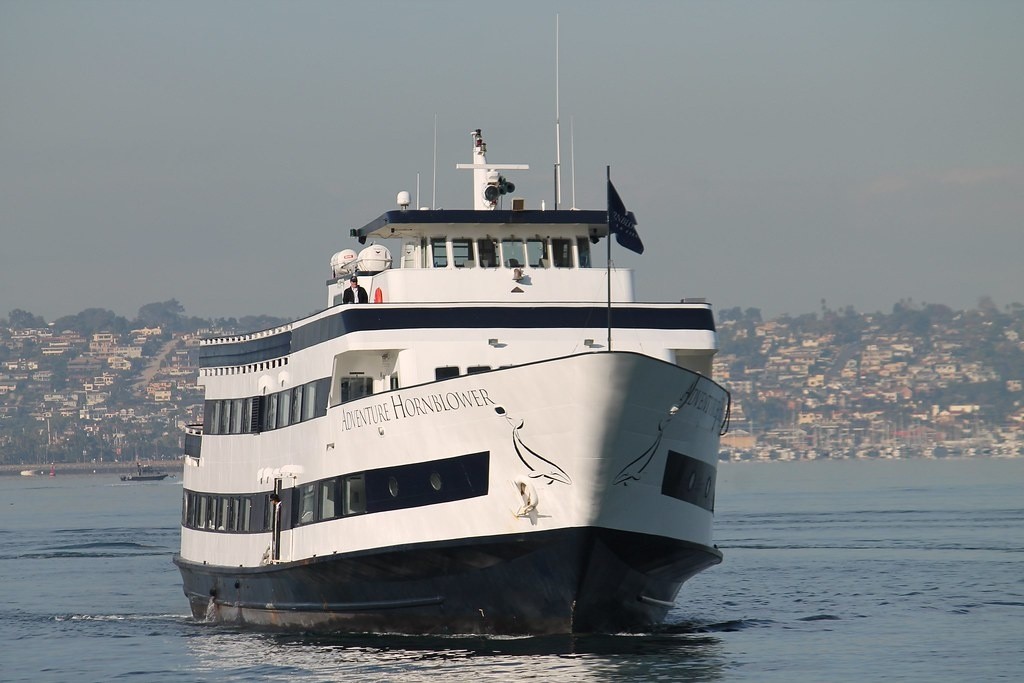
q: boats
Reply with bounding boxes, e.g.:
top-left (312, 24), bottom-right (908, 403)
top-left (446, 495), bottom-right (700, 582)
top-left (174, 130), bottom-right (735, 643)
top-left (119, 464), bottom-right (169, 483)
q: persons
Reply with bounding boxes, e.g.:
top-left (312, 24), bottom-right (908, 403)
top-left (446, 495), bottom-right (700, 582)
top-left (343, 276), bottom-right (368, 304)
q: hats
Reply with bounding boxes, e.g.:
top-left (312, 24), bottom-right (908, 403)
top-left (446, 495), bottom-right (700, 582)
top-left (350, 276), bottom-right (357, 282)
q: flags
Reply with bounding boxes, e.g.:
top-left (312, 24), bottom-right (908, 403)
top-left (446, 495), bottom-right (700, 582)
top-left (607, 178), bottom-right (644, 255)
